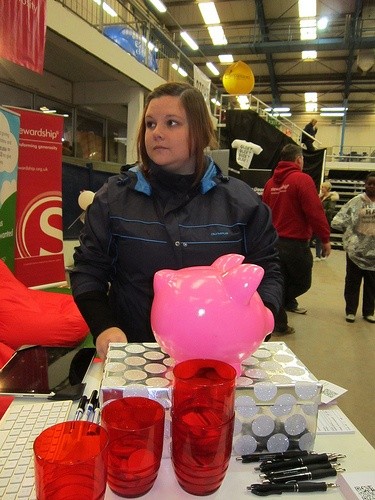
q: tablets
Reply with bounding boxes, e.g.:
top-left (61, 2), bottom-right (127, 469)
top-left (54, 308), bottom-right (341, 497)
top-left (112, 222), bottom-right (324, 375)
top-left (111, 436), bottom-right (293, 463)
top-left (0, 344), bottom-right (97, 398)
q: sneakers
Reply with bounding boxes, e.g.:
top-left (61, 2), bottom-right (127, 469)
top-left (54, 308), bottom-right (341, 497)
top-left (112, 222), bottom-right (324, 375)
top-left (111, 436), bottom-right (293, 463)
top-left (287, 305), bottom-right (307, 314)
top-left (364, 316), bottom-right (375, 323)
top-left (313, 256), bottom-right (326, 262)
top-left (346, 314), bottom-right (355, 322)
top-left (273, 327), bottom-right (296, 336)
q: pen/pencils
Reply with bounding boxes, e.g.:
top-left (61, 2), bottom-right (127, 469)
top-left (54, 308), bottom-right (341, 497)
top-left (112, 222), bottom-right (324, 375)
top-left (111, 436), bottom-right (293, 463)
top-left (236, 451), bottom-right (347, 497)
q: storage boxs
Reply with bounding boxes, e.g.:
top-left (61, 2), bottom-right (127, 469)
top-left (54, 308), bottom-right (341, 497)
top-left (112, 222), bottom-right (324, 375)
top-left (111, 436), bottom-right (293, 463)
top-left (96, 343), bottom-right (324, 460)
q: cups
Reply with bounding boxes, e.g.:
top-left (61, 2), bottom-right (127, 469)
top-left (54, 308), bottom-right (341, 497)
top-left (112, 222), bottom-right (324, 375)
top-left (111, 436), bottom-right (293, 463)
top-left (172, 359), bottom-right (237, 427)
top-left (101, 397), bottom-right (165, 497)
top-left (171, 407), bottom-right (235, 496)
top-left (33, 420), bottom-right (108, 500)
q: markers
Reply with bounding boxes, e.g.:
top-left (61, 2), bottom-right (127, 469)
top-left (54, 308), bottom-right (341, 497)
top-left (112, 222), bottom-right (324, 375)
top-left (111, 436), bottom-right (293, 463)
top-left (87, 403), bottom-right (100, 435)
top-left (69, 396), bottom-right (87, 434)
top-left (84, 390), bottom-right (98, 421)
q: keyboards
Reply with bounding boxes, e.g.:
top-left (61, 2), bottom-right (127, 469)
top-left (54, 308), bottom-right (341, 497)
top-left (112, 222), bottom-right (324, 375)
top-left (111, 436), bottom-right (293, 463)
top-left (0, 399), bottom-right (74, 500)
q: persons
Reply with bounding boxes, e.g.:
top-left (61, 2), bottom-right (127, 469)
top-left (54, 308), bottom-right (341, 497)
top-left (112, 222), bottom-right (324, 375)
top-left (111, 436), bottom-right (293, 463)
top-left (261, 143), bottom-right (332, 337)
top-left (331, 176), bottom-right (374, 324)
top-left (71, 82), bottom-right (284, 362)
top-left (315, 180), bottom-right (337, 263)
top-left (300, 118), bottom-right (320, 151)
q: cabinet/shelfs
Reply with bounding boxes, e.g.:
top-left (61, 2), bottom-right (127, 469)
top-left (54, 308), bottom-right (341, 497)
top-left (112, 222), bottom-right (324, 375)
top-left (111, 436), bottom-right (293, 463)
top-left (324, 179), bottom-right (366, 246)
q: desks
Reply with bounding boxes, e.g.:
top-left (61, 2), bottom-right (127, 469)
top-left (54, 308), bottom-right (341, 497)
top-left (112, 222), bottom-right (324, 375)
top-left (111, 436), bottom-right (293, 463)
top-left (103, 404), bottom-right (375, 500)
